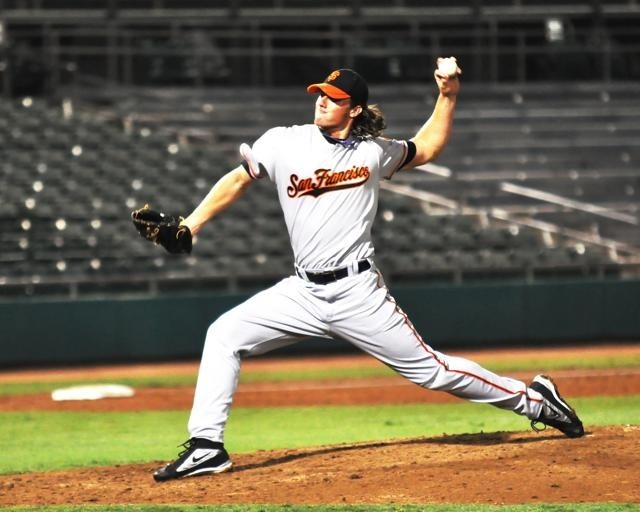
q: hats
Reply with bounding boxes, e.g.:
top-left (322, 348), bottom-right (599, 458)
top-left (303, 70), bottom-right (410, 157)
top-left (308, 68), bottom-right (367, 101)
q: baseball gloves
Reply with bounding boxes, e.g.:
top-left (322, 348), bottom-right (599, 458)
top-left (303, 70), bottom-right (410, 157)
top-left (131, 206), bottom-right (196, 255)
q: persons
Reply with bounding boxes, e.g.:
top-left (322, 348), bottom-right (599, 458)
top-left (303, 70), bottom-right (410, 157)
top-left (129, 52), bottom-right (586, 482)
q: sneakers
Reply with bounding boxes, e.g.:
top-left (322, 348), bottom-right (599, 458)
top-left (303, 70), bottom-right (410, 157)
top-left (154, 437), bottom-right (233, 481)
top-left (528, 375), bottom-right (585, 438)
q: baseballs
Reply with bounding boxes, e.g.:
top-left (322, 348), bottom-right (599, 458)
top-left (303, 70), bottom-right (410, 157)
top-left (438, 58), bottom-right (458, 78)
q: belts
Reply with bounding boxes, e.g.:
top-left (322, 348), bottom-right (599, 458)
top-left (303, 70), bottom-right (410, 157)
top-left (296, 261), bottom-right (372, 283)
